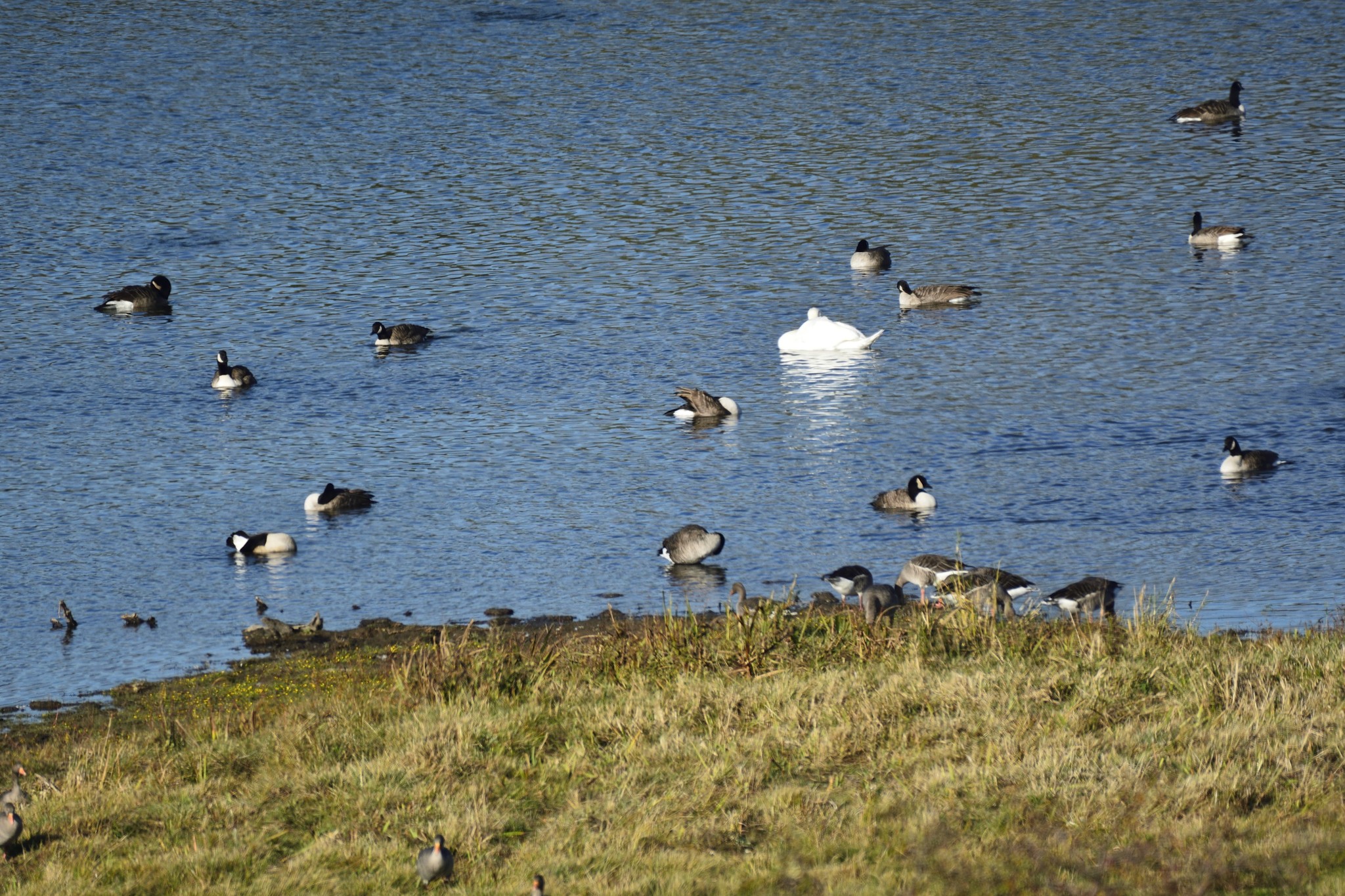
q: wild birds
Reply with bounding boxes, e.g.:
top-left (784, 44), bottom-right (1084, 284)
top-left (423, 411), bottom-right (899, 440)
top-left (1167, 81), bottom-right (1245, 123)
top-left (850, 239), bottom-right (895, 269)
top-left (227, 530), bottom-right (300, 553)
top-left (96, 275), bottom-right (172, 314)
top-left (370, 322), bottom-right (433, 344)
top-left (1189, 212), bottom-right (1255, 243)
top-left (779, 308), bottom-right (885, 350)
top-left (303, 483), bottom-right (378, 509)
top-left (1220, 436), bottom-right (1296, 472)
top-left (663, 386), bottom-right (741, 417)
top-left (212, 350), bottom-right (257, 388)
top-left (656, 474), bottom-right (1124, 630)
top-left (897, 279), bottom-right (983, 305)
